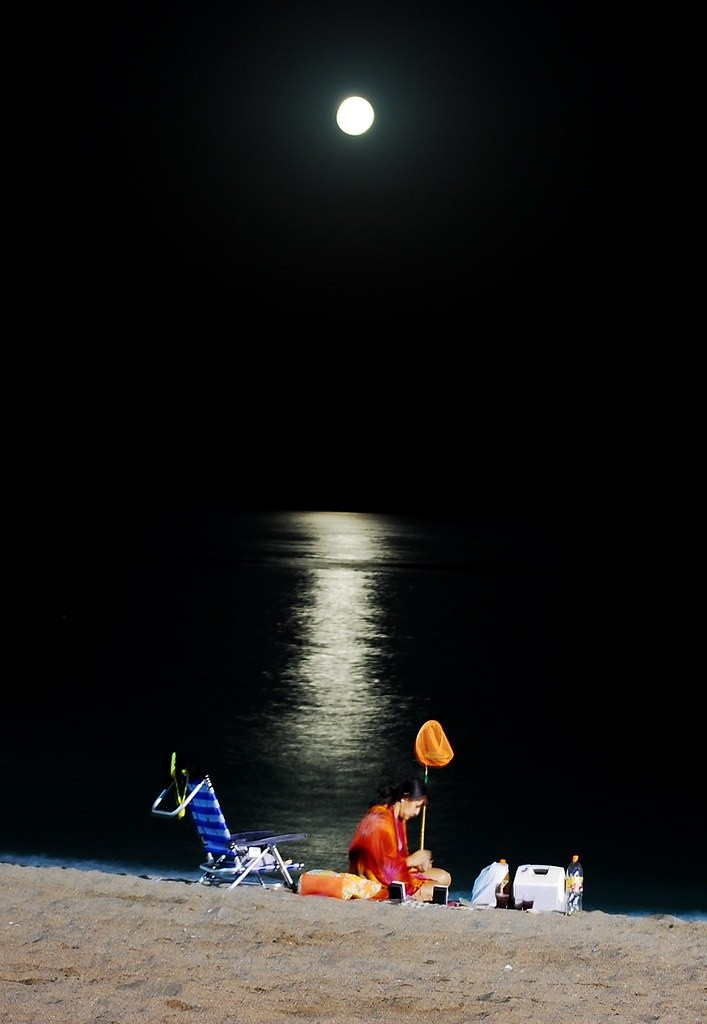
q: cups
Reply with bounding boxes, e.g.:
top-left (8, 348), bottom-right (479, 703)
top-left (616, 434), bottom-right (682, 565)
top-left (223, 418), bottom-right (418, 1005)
top-left (495, 883), bottom-right (510, 909)
top-left (522, 896), bottom-right (534, 910)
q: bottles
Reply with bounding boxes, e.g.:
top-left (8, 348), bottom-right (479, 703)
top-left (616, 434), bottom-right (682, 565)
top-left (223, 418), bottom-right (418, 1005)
top-left (500, 859), bottom-right (509, 881)
top-left (565, 856), bottom-right (583, 914)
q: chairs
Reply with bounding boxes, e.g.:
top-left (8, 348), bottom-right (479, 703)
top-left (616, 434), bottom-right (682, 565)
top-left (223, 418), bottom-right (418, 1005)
top-left (150, 771), bottom-right (309, 893)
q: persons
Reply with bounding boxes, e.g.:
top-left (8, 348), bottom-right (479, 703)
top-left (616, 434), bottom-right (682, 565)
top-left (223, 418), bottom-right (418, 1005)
top-left (348, 778), bottom-right (451, 903)
